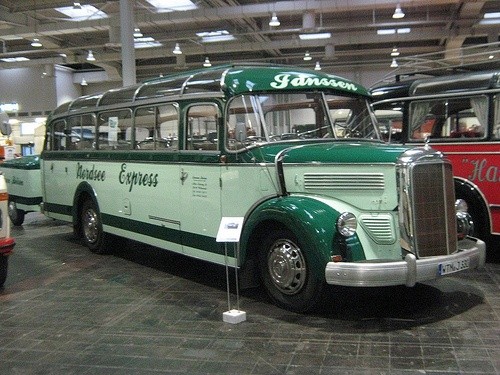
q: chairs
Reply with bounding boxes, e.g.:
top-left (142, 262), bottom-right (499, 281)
top-left (441, 116), bottom-right (458, 137)
top-left (75, 136), bottom-right (209, 150)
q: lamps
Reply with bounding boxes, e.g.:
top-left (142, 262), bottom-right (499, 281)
top-left (30, 0), bottom-right (406, 86)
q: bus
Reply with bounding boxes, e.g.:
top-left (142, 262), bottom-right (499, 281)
top-left (1, 59), bottom-right (484, 297)
top-left (341, 59), bottom-right (500, 249)
